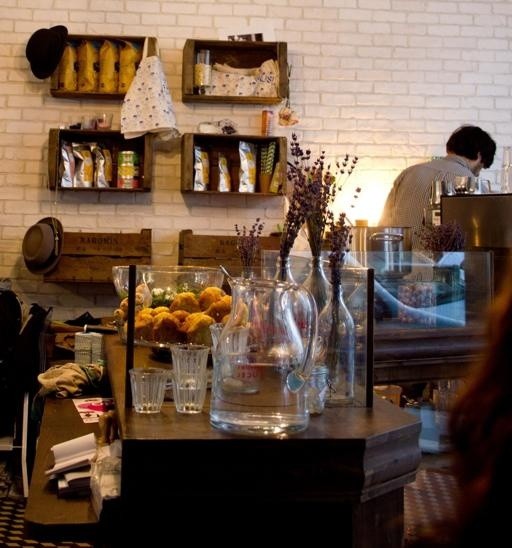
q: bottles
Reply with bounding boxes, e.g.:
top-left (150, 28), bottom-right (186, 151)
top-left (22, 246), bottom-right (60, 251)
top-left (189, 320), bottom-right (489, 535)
top-left (194, 51), bottom-right (212, 96)
top-left (272, 259), bottom-right (357, 407)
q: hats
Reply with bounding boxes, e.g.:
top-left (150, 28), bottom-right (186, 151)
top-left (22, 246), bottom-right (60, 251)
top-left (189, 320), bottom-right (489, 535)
top-left (27, 25), bottom-right (68, 80)
top-left (23, 217), bottom-right (64, 275)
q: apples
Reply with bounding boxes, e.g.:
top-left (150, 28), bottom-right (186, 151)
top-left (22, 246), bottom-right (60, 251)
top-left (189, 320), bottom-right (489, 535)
top-left (114, 287), bottom-right (248, 346)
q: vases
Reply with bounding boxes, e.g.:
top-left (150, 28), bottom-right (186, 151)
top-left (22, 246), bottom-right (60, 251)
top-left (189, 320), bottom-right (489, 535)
top-left (312, 283), bottom-right (356, 408)
top-left (432, 264), bottom-right (467, 328)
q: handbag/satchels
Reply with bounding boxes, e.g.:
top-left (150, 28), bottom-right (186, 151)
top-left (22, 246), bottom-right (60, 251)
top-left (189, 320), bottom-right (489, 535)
top-left (120, 58), bottom-right (179, 142)
top-left (208, 59), bottom-right (279, 98)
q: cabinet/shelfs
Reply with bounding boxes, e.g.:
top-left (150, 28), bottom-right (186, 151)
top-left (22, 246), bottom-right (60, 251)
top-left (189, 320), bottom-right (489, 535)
top-left (50, 36), bottom-right (155, 99)
top-left (179, 132), bottom-right (288, 197)
top-left (181, 37), bottom-right (287, 104)
top-left (47, 127), bottom-right (155, 195)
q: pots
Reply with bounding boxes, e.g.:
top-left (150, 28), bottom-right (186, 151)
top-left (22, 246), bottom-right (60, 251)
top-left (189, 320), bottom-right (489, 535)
top-left (338, 225), bottom-right (413, 276)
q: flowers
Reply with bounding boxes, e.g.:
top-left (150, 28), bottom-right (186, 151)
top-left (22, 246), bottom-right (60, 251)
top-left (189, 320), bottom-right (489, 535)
top-left (414, 219), bottom-right (468, 265)
top-left (229, 133), bottom-right (364, 400)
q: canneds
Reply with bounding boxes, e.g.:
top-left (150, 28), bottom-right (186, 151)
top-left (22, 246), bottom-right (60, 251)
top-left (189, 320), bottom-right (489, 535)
top-left (117, 151), bottom-right (140, 189)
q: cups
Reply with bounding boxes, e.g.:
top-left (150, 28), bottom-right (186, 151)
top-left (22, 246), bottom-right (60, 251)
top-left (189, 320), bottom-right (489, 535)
top-left (426, 176), bottom-right (481, 208)
top-left (130, 341), bottom-right (210, 415)
top-left (95, 114), bottom-right (113, 130)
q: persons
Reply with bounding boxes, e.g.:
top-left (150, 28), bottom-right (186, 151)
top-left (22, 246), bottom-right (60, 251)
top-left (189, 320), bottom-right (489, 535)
top-left (444, 270), bottom-right (512, 547)
top-left (380, 125), bottom-right (496, 281)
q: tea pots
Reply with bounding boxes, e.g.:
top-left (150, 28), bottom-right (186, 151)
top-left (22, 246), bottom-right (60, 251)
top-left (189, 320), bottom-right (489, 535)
top-left (213, 275), bottom-right (311, 436)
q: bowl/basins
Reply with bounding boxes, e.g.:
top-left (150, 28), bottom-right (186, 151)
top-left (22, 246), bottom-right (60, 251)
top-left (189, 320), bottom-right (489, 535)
top-left (112, 265), bottom-right (224, 301)
